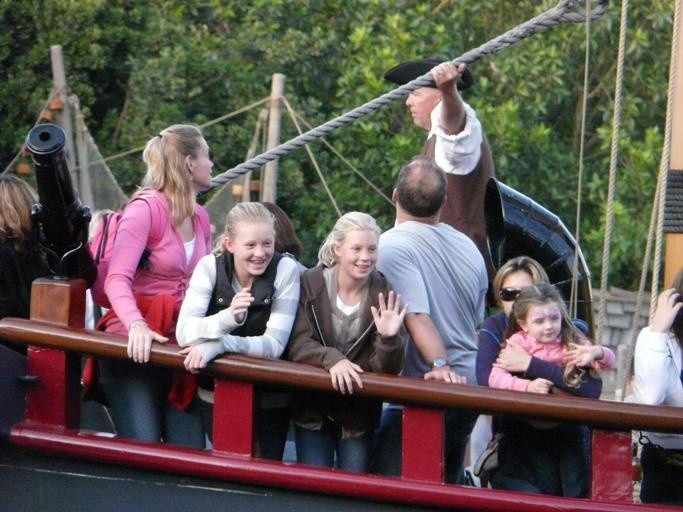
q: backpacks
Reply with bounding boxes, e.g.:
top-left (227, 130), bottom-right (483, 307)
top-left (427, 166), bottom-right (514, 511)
top-left (86, 190), bottom-right (211, 309)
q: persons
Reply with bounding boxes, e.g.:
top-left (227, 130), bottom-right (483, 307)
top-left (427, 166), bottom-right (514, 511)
top-left (372, 157), bottom-right (491, 484)
top-left (474, 255), bottom-right (604, 502)
top-left (384, 56), bottom-right (508, 309)
top-left (172, 201), bottom-right (301, 463)
top-left (92, 122), bottom-right (215, 451)
top-left (284, 211), bottom-right (411, 479)
top-left (1, 173), bottom-right (50, 360)
top-left (261, 203), bottom-right (309, 273)
top-left (620, 269), bottom-right (683, 504)
top-left (489, 283), bottom-right (618, 496)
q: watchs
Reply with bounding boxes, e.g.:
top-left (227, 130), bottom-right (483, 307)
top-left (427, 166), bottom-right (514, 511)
top-left (431, 357), bottom-right (449, 368)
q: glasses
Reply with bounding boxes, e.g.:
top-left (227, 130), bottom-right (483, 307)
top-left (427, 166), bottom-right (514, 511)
top-left (499, 287), bottom-right (523, 303)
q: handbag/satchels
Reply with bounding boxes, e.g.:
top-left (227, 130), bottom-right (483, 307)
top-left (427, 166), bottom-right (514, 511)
top-left (473, 434), bottom-right (499, 479)
top-left (637, 435), bottom-right (683, 508)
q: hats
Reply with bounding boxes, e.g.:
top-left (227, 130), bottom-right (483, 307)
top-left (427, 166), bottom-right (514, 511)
top-left (383, 58), bottom-right (474, 92)
